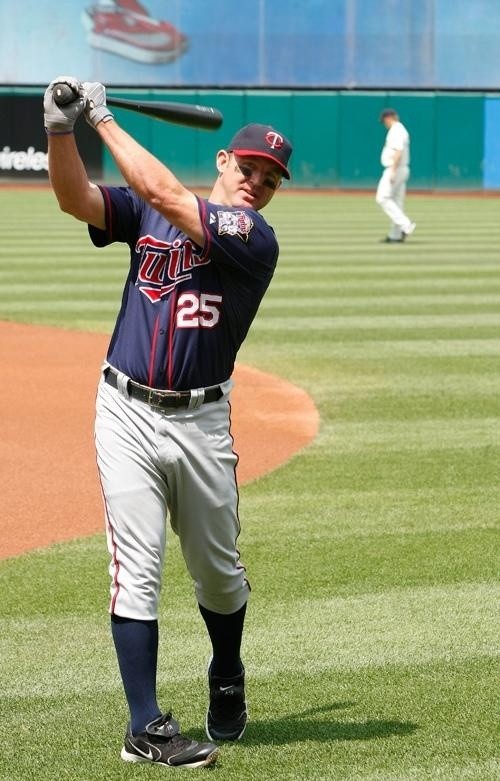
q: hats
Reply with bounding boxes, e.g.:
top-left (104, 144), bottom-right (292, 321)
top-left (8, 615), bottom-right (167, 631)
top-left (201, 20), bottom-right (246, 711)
top-left (224, 123), bottom-right (294, 182)
top-left (380, 109), bottom-right (397, 124)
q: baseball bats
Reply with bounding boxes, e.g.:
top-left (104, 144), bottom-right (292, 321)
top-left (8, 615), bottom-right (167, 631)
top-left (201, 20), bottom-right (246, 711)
top-left (52, 85), bottom-right (224, 129)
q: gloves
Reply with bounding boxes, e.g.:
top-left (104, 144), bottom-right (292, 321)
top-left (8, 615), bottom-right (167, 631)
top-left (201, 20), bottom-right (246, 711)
top-left (83, 82), bottom-right (113, 129)
top-left (42, 76), bottom-right (86, 127)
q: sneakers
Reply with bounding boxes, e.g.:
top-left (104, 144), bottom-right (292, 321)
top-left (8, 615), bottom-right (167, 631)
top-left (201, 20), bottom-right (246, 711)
top-left (383, 223), bottom-right (417, 244)
top-left (82, 1), bottom-right (188, 64)
top-left (204, 654), bottom-right (249, 746)
top-left (120, 711), bottom-right (221, 771)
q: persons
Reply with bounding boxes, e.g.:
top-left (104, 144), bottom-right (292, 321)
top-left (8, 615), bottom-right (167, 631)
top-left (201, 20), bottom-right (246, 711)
top-left (43, 77), bottom-right (293, 770)
top-left (375, 109), bottom-right (417, 243)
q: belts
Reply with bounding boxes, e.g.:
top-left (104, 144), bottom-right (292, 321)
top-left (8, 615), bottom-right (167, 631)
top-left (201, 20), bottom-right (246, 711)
top-left (104, 367), bottom-right (224, 411)
